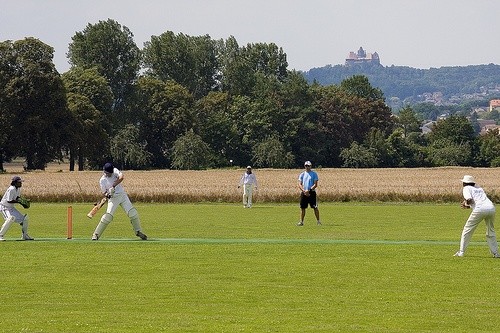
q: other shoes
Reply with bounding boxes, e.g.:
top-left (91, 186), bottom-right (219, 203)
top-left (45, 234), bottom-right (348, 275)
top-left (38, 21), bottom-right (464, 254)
top-left (316, 221), bottom-right (322, 225)
top-left (0, 236), bottom-right (6, 241)
top-left (452, 252), bottom-right (465, 257)
top-left (297, 222), bottom-right (303, 226)
top-left (136, 231), bottom-right (147, 240)
top-left (92, 235), bottom-right (99, 241)
top-left (493, 252), bottom-right (500, 258)
top-left (22, 235), bottom-right (34, 240)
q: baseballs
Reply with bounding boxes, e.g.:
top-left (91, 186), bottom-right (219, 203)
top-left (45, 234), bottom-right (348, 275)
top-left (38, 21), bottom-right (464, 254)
top-left (229, 159), bottom-right (233, 163)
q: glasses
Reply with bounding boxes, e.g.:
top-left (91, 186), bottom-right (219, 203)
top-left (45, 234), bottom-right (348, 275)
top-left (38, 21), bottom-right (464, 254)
top-left (305, 165), bottom-right (310, 167)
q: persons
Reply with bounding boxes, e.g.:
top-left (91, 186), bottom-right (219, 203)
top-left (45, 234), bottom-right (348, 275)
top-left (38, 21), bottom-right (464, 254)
top-left (92, 163), bottom-right (147, 241)
top-left (296, 161), bottom-right (322, 226)
top-left (238, 166), bottom-right (258, 208)
top-left (0, 176), bottom-right (34, 240)
top-left (452, 175), bottom-right (500, 258)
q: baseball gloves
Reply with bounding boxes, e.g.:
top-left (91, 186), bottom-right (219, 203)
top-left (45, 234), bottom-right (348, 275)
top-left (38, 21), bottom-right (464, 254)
top-left (17, 194), bottom-right (31, 208)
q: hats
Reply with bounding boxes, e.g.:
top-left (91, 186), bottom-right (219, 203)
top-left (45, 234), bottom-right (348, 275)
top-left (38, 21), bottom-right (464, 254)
top-left (247, 166), bottom-right (251, 168)
top-left (304, 161), bottom-right (312, 165)
top-left (104, 163), bottom-right (114, 173)
top-left (460, 175), bottom-right (476, 184)
top-left (11, 176), bottom-right (24, 182)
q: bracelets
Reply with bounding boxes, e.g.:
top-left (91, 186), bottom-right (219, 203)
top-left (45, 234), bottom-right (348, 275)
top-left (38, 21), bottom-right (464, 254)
top-left (309, 189), bottom-right (312, 192)
top-left (113, 185), bottom-right (115, 188)
